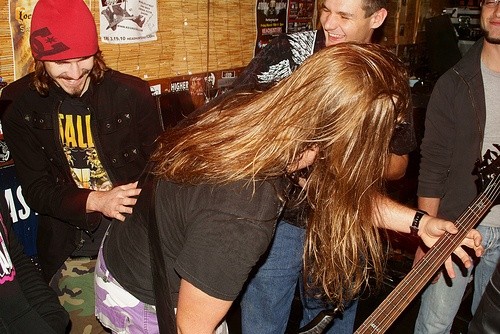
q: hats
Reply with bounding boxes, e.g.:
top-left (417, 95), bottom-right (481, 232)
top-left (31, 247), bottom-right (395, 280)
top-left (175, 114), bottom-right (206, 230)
top-left (30, 0), bottom-right (98, 61)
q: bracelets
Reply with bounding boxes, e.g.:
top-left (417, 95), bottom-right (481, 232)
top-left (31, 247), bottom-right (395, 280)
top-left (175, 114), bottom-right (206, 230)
top-left (410, 210), bottom-right (428, 238)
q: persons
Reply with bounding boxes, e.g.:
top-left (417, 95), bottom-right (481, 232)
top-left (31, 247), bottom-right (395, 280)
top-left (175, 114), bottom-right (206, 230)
top-left (225, 0), bottom-right (412, 334)
top-left (0, 0), bottom-right (162, 334)
top-left (94, 43), bottom-right (483, 333)
top-left (415, 0), bottom-right (499, 334)
top-left (1, 195), bottom-right (72, 334)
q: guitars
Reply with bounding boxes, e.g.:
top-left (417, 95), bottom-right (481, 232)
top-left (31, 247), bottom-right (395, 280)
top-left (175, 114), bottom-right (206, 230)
top-left (352, 144), bottom-right (500, 334)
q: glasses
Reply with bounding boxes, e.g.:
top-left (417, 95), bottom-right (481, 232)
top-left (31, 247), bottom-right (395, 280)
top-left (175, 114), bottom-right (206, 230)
top-left (482, 0), bottom-right (500, 8)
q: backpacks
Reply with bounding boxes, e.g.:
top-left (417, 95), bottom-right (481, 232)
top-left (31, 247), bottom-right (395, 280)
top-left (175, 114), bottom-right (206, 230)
top-left (420, 14), bottom-right (463, 73)
